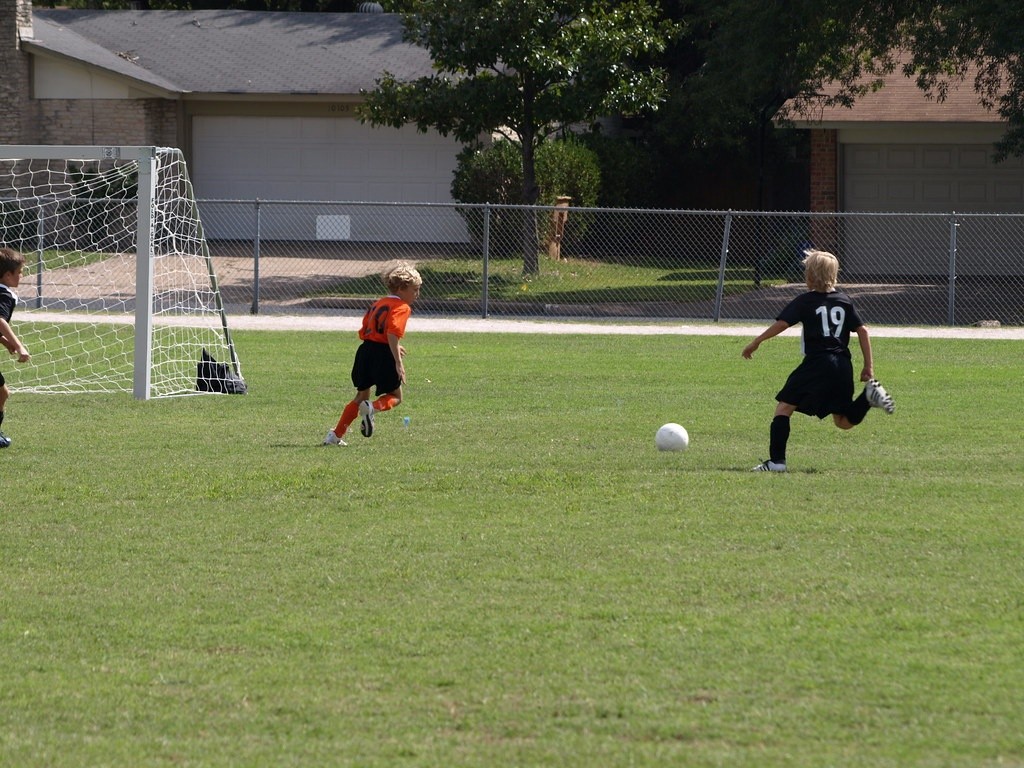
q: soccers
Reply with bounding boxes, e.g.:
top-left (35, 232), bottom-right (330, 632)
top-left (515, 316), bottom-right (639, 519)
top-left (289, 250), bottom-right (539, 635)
top-left (655, 422), bottom-right (689, 451)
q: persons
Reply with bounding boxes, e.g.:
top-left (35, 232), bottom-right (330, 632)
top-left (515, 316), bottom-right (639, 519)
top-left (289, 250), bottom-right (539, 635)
top-left (323, 264), bottom-right (422, 446)
top-left (742, 250), bottom-right (896, 472)
top-left (0, 247), bottom-right (31, 447)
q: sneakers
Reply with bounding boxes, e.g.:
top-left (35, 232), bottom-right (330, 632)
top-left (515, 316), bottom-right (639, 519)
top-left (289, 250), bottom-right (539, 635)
top-left (0, 431), bottom-right (11, 448)
top-left (866, 379), bottom-right (896, 414)
top-left (323, 429), bottom-right (348, 445)
top-left (358, 399), bottom-right (375, 438)
top-left (748, 456), bottom-right (788, 473)
